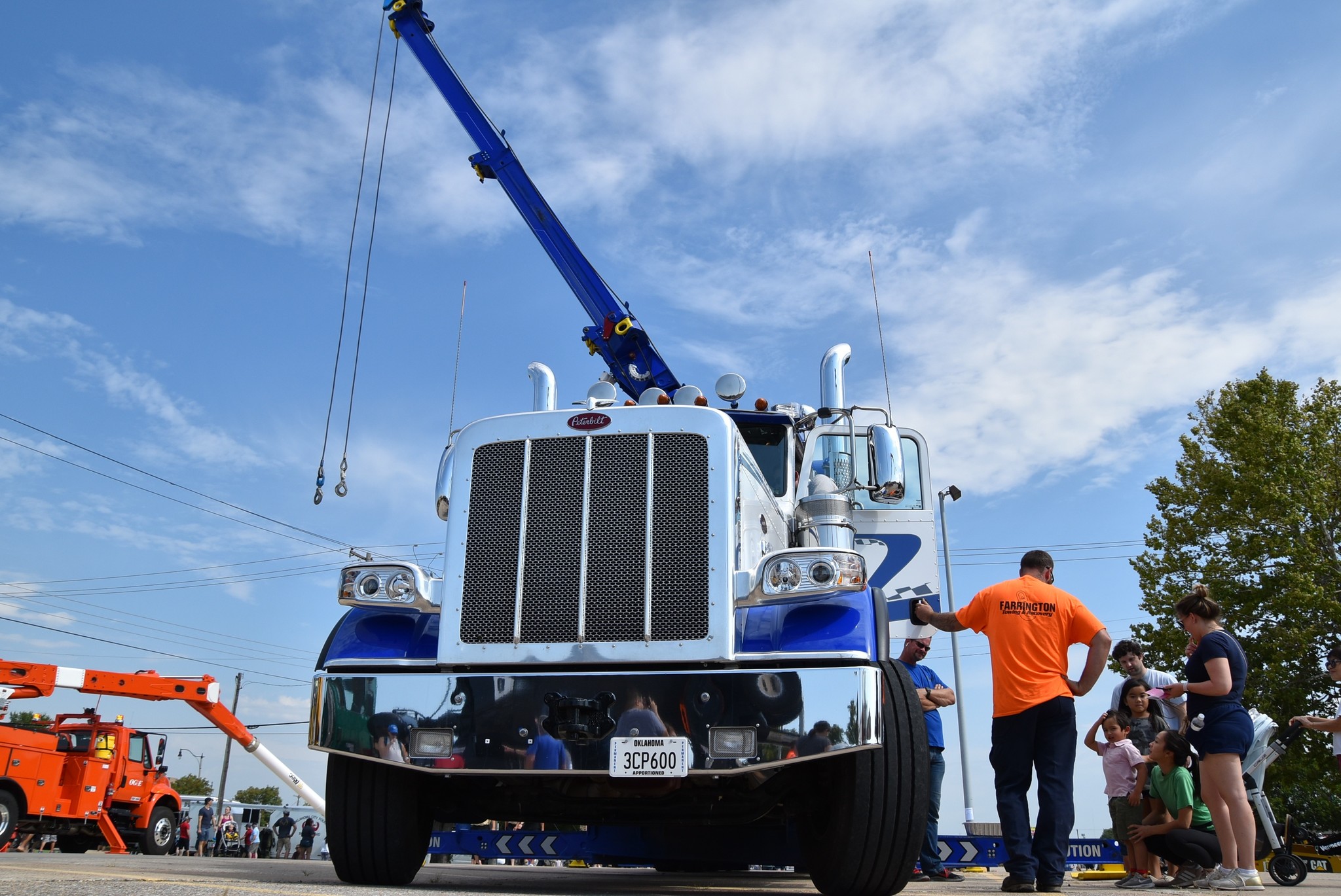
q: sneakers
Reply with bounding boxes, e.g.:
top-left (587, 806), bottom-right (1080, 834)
top-left (1207, 868), bottom-right (1265, 890)
top-left (908, 868), bottom-right (931, 882)
top-left (1114, 871), bottom-right (1136, 887)
top-left (929, 868), bottom-right (965, 882)
top-left (1167, 866), bottom-right (1199, 887)
top-left (1193, 868), bottom-right (1229, 888)
top-left (1122, 872), bottom-right (1155, 888)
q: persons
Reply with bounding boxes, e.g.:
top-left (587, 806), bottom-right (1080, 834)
top-left (272, 810), bottom-right (296, 859)
top-left (883, 636), bottom-right (965, 882)
top-left (470, 817), bottom-right (602, 868)
top-left (378, 725), bottom-right (410, 764)
top-left (615, 690), bottom-right (669, 737)
top-left (240, 822), bottom-right (260, 858)
top-left (194, 797), bottom-right (216, 857)
top-left (16, 833), bottom-right (57, 853)
top-left (321, 834), bottom-right (331, 861)
top-left (175, 816), bottom-right (192, 856)
top-left (132, 850), bottom-right (140, 855)
top-left (1287, 645), bottom-right (1341, 779)
top-left (795, 721), bottom-right (831, 757)
top-left (259, 822), bottom-right (275, 859)
top-left (502, 715), bottom-right (572, 770)
top-left (214, 806), bottom-right (239, 857)
top-left (1084, 586), bottom-right (1266, 890)
top-left (292, 818), bottom-right (316, 860)
top-left (97, 845), bottom-right (106, 851)
top-left (916, 551), bottom-right (1111, 892)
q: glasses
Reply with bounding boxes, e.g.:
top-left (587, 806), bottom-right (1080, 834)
top-left (1325, 659), bottom-right (1341, 671)
top-left (391, 733), bottom-right (398, 736)
top-left (910, 638), bottom-right (930, 651)
top-left (295, 847), bottom-right (300, 849)
top-left (1177, 612), bottom-right (1198, 629)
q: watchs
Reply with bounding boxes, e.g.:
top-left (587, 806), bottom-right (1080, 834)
top-left (924, 687), bottom-right (931, 700)
top-left (1183, 683), bottom-right (1191, 694)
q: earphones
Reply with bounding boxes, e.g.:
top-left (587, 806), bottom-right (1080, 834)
top-left (906, 642), bottom-right (909, 646)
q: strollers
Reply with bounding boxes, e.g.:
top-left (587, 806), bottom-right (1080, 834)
top-left (213, 820), bottom-right (241, 858)
top-left (1239, 708), bottom-right (1308, 887)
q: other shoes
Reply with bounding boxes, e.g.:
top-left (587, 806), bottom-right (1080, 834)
top-left (1153, 875), bottom-right (1175, 887)
top-left (1148, 875), bottom-right (1158, 883)
top-left (528, 864), bottom-right (534, 866)
top-left (1035, 881), bottom-right (1063, 892)
top-left (1001, 876), bottom-right (1034, 892)
top-left (49, 851), bottom-right (54, 853)
top-left (38, 851), bottom-right (43, 853)
top-left (15, 849), bottom-right (27, 852)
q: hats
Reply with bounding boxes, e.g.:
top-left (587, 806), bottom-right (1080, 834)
top-left (388, 724), bottom-right (398, 734)
top-left (244, 824), bottom-right (251, 827)
top-left (183, 816), bottom-right (192, 821)
top-left (283, 810), bottom-right (290, 814)
top-left (251, 822), bottom-right (258, 826)
top-left (204, 798), bottom-right (211, 805)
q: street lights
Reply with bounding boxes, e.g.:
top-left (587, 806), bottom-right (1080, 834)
top-left (178, 748), bottom-right (205, 778)
top-left (937, 485), bottom-right (974, 823)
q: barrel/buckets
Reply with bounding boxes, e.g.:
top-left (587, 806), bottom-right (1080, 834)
top-left (94, 732), bottom-right (116, 759)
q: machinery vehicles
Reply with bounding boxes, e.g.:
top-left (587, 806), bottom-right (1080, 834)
top-left (306, 2), bottom-right (942, 896)
top-left (0, 657), bottom-right (325, 863)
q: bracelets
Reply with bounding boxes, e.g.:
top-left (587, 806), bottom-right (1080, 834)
top-left (1311, 723), bottom-right (1315, 729)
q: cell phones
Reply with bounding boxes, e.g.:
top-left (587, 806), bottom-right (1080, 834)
top-left (646, 696), bottom-right (651, 708)
top-left (1156, 686), bottom-right (1172, 690)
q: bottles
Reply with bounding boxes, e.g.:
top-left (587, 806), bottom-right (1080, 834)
top-left (1190, 713), bottom-right (1205, 731)
